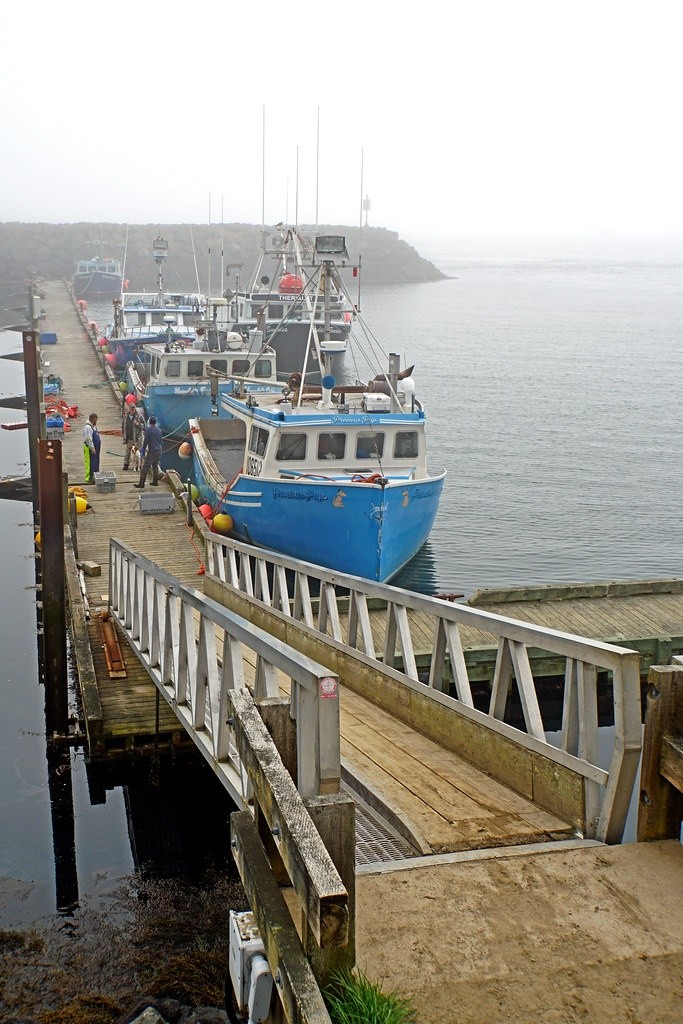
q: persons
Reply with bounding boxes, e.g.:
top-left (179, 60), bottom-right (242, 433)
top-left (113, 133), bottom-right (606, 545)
top-left (134, 417), bottom-right (162, 488)
top-left (122, 403), bottom-right (146, 470)
top-left (83, 413), bottom-right (101, 483)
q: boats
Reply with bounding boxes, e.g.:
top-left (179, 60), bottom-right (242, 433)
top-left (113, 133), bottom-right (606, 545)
top-left (125, 190), bottom-right (294, 460)
top-left (99, 227), bottom-right (210, 374)
top-left (187, 102), bottom-right (450, 585)
top-left (74, 253), bottom-right (128, 295)
top-left (218, 193), bottom-right (353, 378)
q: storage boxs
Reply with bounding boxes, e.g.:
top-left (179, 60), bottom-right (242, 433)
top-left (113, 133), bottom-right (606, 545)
top-left (208, 298), bottom-right (227, 304)
top-left (138, 492), bottom-right (175, 515)
top-left (39, 333), bottom-right (56, 344)
top-left (81, 560), bottom-right (101, 577)
top-left (362, 392), bottom-right (390, 413)
top-left (46, 418), bottom-right (65, 440)
top-left (94, 471), bottom-right (117, 492)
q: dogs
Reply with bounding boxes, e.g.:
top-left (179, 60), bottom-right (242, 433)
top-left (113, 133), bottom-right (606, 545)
top-left (127, 445), bottom-right (142, 472)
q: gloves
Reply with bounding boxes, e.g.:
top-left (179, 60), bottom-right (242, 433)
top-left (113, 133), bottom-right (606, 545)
top-left (141, 448), bottom-right (146, 459)
top-left (90, 447), bottom-right (96, 455)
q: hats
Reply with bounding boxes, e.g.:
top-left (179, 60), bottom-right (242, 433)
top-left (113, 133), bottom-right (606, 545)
top-left (130, 403), bottom-right (136, 408)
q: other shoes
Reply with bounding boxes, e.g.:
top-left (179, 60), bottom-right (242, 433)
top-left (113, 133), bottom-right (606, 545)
top-left (123, 465), bottom-right (128, 470)
top-left (150, 482), bottom-right (158, 485)
top-left (134, 484), bottom-right (144, 489)
